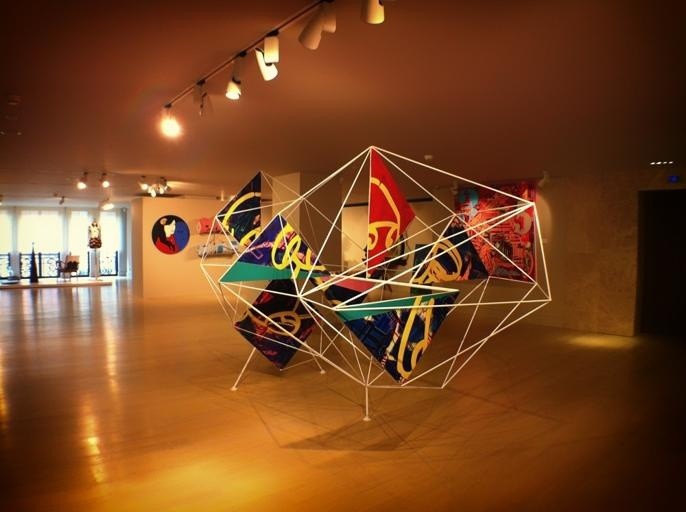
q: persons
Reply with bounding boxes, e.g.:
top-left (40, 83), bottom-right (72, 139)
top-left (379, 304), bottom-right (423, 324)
top-left (154, 216), bottom-right (180, 253)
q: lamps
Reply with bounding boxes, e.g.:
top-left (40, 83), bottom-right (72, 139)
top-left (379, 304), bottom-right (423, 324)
top-left (75, 170), bottom-right (171, 197)
top-left (154, 1), bottom-right (386, 135)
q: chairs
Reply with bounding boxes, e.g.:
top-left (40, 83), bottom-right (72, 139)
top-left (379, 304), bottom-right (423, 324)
top-left (54, 255), bottom-right (79, 282)
